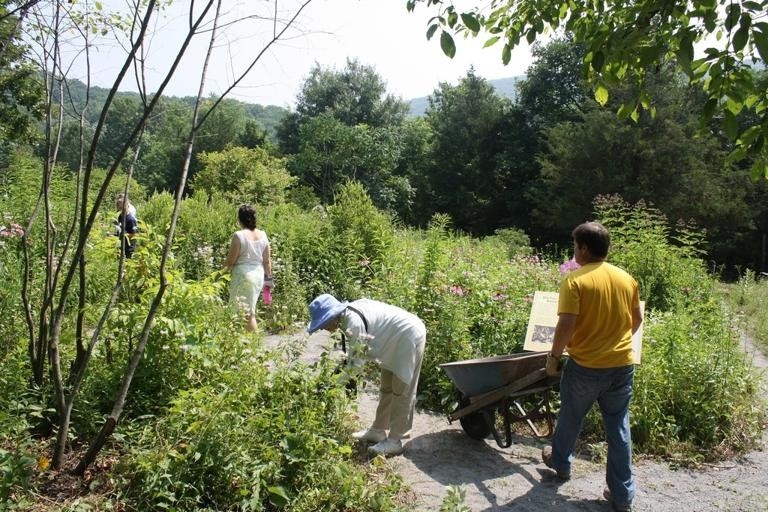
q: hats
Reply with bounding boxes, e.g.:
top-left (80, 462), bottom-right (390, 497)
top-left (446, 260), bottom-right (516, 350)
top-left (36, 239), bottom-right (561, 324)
top-left (307, 293), bottom-right (349, 334)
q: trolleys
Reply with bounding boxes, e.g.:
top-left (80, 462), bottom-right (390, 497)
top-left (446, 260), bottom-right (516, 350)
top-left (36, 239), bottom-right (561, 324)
top-left (438, 349), bottom-right (563, 447)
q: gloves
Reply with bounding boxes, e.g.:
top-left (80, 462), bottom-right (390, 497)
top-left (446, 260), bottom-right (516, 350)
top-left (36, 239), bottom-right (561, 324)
top-left (263, 274), bottom-right (276, 288)
top-left (545, 352), bottom-right (562, 377)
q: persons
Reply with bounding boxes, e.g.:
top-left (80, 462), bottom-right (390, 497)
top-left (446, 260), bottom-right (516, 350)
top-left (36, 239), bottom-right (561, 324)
top-left (541, 222), bottom-right (644, 511)
top-left (306, 292), bottom-right (427, 457)
top-left (111, 191), bottom-right (137, 226)
top-left (105, 197), bottom-right (137, 260)
top-left (221, 206), bottom-right (274, 334)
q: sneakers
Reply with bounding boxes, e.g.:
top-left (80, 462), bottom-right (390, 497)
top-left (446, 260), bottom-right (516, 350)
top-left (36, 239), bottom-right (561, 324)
top-left (368, 438), bottom-right (403, 456)
top-left (603, 486), bottom-right (634, 512)
top-left (542, 445), bottom-right (572, 478)
top-left (350, 426), bottom-right (387, 442)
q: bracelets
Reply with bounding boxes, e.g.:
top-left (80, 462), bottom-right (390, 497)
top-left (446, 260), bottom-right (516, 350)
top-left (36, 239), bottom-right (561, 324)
top-left (547, 350), bottom-right (559, 360)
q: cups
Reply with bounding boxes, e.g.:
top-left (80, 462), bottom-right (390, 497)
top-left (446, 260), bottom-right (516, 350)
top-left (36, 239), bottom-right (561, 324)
top-left (262, 286), bottom-right (271, 305)
